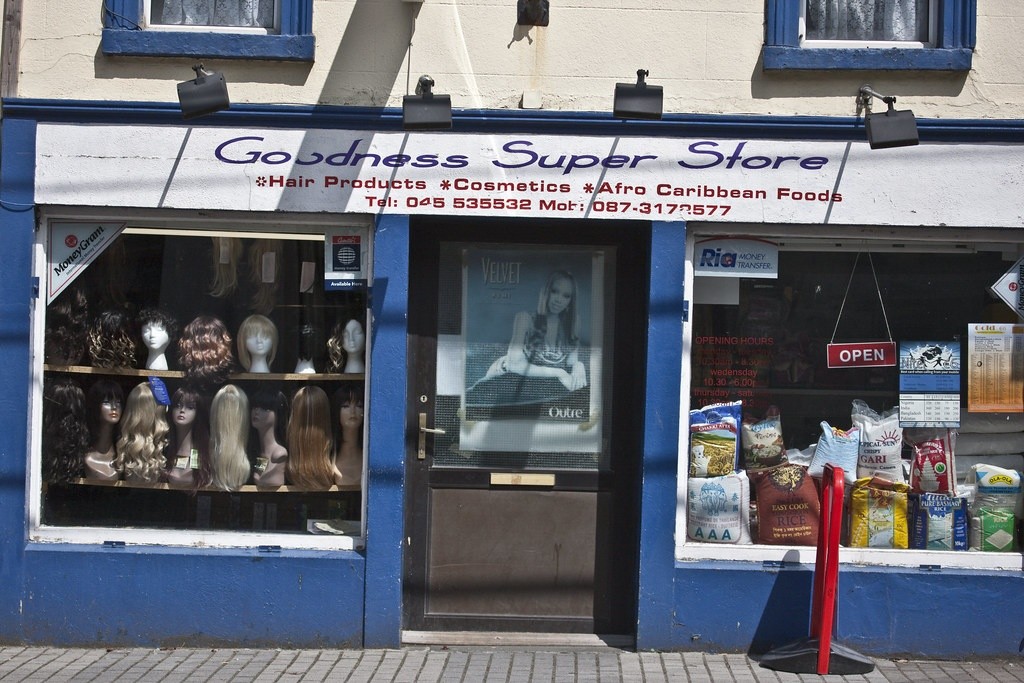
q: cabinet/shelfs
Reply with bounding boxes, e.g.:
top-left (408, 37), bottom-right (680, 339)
top-left (42, 365), bottom-right (364, 491)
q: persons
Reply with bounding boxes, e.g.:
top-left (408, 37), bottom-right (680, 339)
top-left (43, 291), bottom-right (366, 491)
top-left (466, 270), bottom-right (586, 417)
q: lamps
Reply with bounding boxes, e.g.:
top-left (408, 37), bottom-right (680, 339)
top-left (613, 69), bottom-right (663, 120)
top-left (860, 84), bottom-right (919, 150)
top-left (177, 62), bottom-right (230, 119)
top-left (402, 76), bottom-right (453, 129)
